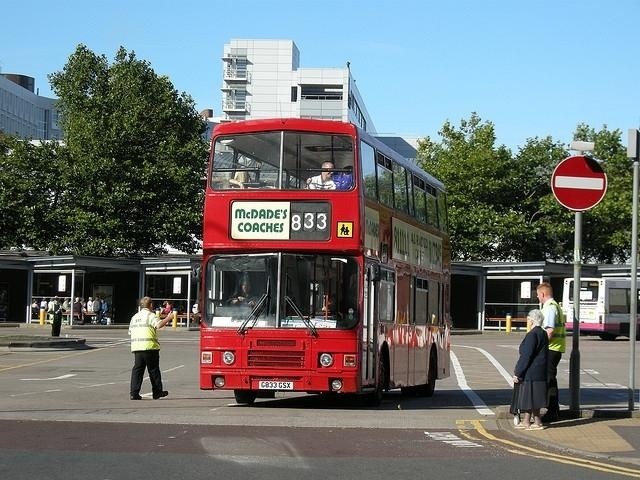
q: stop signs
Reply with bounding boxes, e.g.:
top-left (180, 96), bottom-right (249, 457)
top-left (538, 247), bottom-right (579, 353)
top-left (551, 155), bottom-right (609, 211)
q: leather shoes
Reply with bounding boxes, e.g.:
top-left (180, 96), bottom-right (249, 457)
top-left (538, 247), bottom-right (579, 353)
top-left (130, 395), bottom-right (141, 400)
top-left (153, 391), bottom-right (168, 399)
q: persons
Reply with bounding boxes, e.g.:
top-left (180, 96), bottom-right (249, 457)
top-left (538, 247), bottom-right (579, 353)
top-left (338, 164), bottom-right (354, 190)
top-left (302, 161), bottom-right (336, 190)
top-left (127, 296), bottom-right (174, 400)
top-left (230, 281), bottom-right (263, 306)
top-left (161, 296), bottom-right (174, 313)
top-left (509, 309), bottom-right (553, 430)
top-left (191, 299), bottom-right (201, 315)
top-left (536, 282), bottom-right (566, 422)
top-left (31, 295), bottom-right (109, 325)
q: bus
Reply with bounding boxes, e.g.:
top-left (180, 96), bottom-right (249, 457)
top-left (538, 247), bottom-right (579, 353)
top-left (199, 117), bottom-right (451, 403)
top-left (562, 278), bottom-right (640, 340)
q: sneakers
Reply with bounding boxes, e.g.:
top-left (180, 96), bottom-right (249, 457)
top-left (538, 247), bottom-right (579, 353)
top-left (515, 414), bottom-right (559, 430)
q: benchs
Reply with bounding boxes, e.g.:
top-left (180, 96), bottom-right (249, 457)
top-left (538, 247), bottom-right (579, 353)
top-left (160, 312), bottom-right (201, 326)
top-left (61, 311), bottom-right (98, 325)
